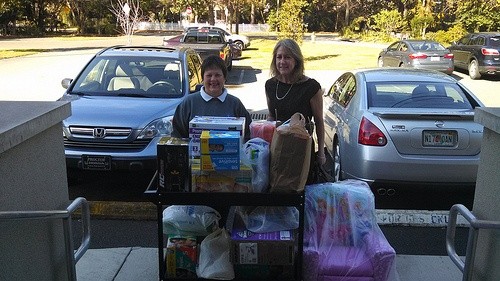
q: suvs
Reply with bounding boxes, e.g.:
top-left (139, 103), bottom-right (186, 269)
top-left (55, 43), bottom-right (204, 181)
top-left (445, 32), bottom-right (500, 80)
top-left (161, 26), bottom-right (250, 75)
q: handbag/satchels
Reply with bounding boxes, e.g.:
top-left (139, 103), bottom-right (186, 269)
top-left (270, 112), bottom-right (313, 195)
top-left (195, 225), bottom-right (236, 281)
top-left (243, 136), bottom-right (270, 194)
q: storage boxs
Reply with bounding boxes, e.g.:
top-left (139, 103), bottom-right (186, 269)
top-left (229, 220), bottom-right (294, 266)
top-left (200, 130), bottom-right (240, 170)
top-left (189, 116), bottom-right (245, 160)
top-left (166, 234), bottom-right (196, 267)
top-left (157, 136), bottom-right (192, 192)
top-left (191, 159), bottom-right (253, 193)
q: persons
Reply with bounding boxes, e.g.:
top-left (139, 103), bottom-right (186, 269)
top-left (171, 55), bottom-right (252, 141)
top-left (265, 39), bottom-right (326, 185)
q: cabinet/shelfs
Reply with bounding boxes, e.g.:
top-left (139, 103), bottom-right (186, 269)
top-left (144, 168), bottom-right (305, 281)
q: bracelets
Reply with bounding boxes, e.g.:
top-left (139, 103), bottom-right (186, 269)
top-left (317, 154), bottom-right (325, 159)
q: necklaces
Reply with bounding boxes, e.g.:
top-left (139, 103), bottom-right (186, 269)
top-left (276, 78), bottom-right (294, 99)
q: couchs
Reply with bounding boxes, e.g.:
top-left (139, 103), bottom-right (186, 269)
top-left (294, 179), bottom-right (396, 281)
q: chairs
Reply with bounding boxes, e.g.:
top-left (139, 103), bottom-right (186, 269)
top-left (412, 86), bottom-right (429, 97)
top-left (107, 64), bottom-right (140, 91)
top-left (159, 64), bottom-right (181, 92)
top-left (370, 85), bottom-right (383, 106)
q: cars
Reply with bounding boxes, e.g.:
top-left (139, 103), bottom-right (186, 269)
top-left (311, 67), bottom-right (489, 211)
top-left (378, 39), bottom-right (455, 76)
